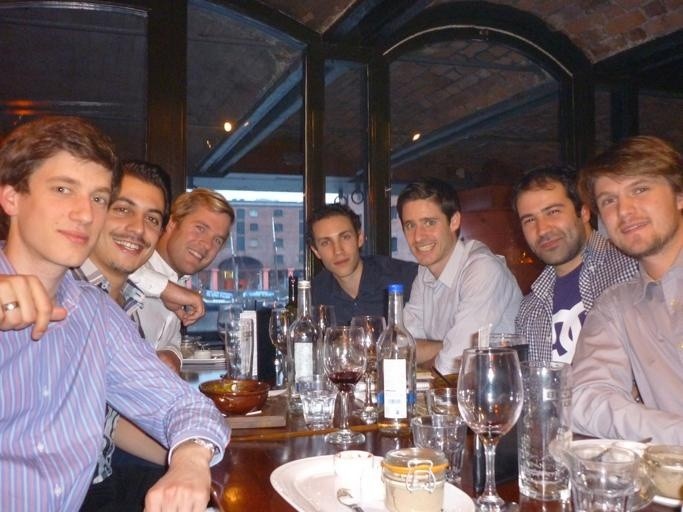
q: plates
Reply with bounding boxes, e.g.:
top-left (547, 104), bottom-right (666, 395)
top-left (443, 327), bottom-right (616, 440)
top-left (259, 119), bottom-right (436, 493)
top-left (181, 350), bottom-right (226, 365)
top-left (270, 454), bottom-right (476, 512)
top-left (569, 438), bottom-right (646, 455)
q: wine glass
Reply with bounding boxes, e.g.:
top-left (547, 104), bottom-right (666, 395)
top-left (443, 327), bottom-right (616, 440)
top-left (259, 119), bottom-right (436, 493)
top-left (323, 324), bottom-right (367, 446)
top-left (350, 313), bottom-right (386, 418)
top-left (269, 307), bottom-right (298, 397)
top-left (457, 347), bottom-right (524, 512)
top-left (217, 303), bottom-right (244, 378)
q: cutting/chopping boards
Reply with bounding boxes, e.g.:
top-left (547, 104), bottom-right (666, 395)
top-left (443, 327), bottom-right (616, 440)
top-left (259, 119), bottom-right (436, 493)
top-left (224, 394), bottom-right (288, 429)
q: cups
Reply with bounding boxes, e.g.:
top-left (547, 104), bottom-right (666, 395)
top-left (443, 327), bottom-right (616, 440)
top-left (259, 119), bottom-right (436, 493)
top-left (195, 350), bottom-right (211, 360)
top-left (225, 318), bottom-right (254, 380)
top-left (516, 360), bottom-right (577, 502)
top-left (567, 442), bottom-right (636, 512)
top-left (411, 415), bottom-right (463, 483)
top-left (336, 450), bottom-right (375, 489)
top-left (643, 444), bottom-right (683, 501)
top-left (477, 322), bottom-right (529, 491)
top-left (423, 388), bottom-right (467, 475)
top-left (297, 374), bottom-right (339, 432)
top-left (382, 444), bottom-right (451, 512)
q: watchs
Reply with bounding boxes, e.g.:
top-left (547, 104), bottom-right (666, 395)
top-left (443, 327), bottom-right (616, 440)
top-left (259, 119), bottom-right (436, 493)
top-left (192, 435), bottom-right (216, 454)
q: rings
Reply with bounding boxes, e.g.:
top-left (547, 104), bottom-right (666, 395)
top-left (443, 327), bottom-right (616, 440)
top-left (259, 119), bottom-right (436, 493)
top-left (1, 299), bottom-right (20, 312)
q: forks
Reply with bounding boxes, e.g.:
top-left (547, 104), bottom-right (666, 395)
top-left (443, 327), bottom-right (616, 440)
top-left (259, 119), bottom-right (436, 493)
top-left (336, 487), bottom-right (365, 512)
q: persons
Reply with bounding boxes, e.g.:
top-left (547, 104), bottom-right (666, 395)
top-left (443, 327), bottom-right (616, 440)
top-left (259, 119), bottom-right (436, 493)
top-left (513, 162), bottom-right (640, 425)
top-left (128, 188), bottom-right (235, 376)
top-left (396, 178), bottom-right (529, 492)
top-left (0, 114), bottom-right (233, 511)
top-left (310, 203), bottom-right (418, 343)
top-left (565, 137), bottom-right (683, 451)
top-left (71, 157), bottom-right (171, 511)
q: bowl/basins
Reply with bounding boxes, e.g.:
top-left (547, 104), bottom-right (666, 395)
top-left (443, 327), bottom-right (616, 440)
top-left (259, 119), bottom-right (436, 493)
top-left (199, 379), bottom-right (271, 415)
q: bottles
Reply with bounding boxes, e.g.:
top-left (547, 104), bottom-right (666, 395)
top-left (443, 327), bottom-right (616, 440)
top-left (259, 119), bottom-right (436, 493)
top-left (316, 302), bottom-right (331, 335)
top-left (180, 337), bottom-right (195, 357)
top-left (286, 279), bottom-right (323, 416)
top-left (285, 275), bottom-right (299, 326)
top-left (376, 284), bottom-right (417, 438)
